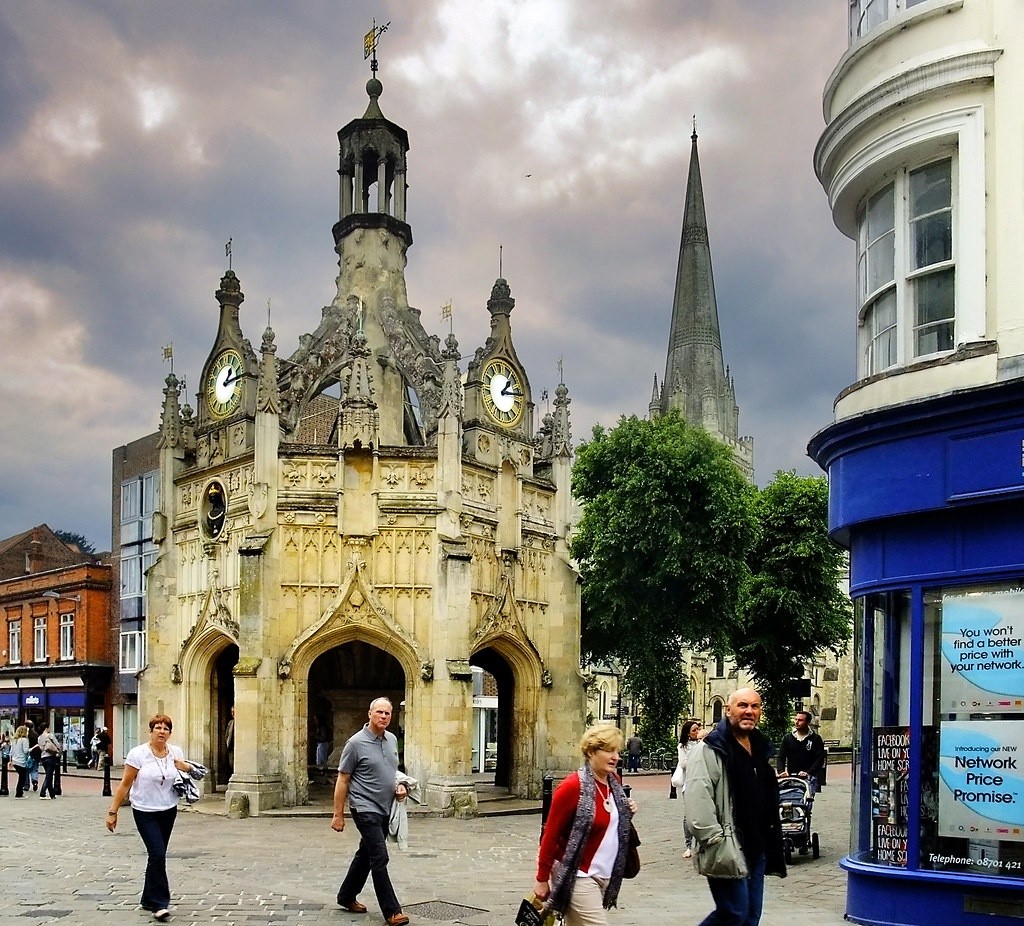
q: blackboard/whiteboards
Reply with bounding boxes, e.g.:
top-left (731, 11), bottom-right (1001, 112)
top-left (874, 725), bottom-right (968, 871)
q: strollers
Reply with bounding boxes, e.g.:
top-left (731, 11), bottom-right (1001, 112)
top-left (776, 773), bottom-right (819, 865)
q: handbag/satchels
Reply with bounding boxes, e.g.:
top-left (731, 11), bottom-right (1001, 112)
top-left (622, 822), bottom-right (642, 880)
top-left (513, 894), bottom-right (556, 926)
top-left (44, 733), bottom-right (60, 755)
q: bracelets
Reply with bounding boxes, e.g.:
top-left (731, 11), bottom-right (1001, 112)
top-left (109, 812), bottom-right (116, 816)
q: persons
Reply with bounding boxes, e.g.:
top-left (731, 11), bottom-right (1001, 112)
top-left (626, 732), bottom-right (644, 772)
top-left (105, 715), bottom-right (191, 920)
top-left (534, 725), bottom-right (638, 926)
top-left (224, 705), bottom-right (234, 767)
top-left (37, 722), bottom-right (61, 800)
top-left (87, 727), bottom-right (110, 770)
top-left (331, 695), bottom-right (409, 926)
top-left (9, 719), bottom-right (41, 800)
top-left (776, 712), bottom-right (824, 815)
top-left (312, 714), bottom-right (329, 764)
top-left (672, 722), bottom-right (711, 859)
top-left (682, 688), bottom-right (787, 926)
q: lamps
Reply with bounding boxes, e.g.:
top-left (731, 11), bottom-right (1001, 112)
top-left (42, 591), bottom-right (81, 604)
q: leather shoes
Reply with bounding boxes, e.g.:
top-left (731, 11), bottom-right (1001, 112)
top-left (346, 899), bottom-right (366, 913)
top-left (387, 910), bottom-right (409, 926)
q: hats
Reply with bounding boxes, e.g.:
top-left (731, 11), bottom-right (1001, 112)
top-left (103, 727), bottom-right (109, 731)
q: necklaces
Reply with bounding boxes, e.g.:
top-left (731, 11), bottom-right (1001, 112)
top-left (595, 782), bottom-right (613, 813)
top-left (151, 745), bottom-right (167, 784)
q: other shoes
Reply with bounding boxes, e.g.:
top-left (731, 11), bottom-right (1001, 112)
top-left (0, 793), bottom-right (9, 796)
top-left (39, 796), bottom-right (49, 800)
top-left (51, 795), bottom-right (57, 800)
top-left (86, 764), bottom-right (91, 769)
top-left (681, 848), bottom-right (692, 858)
top-left (154, 908), bottom-right (169, 920)
top-left (14, 795), bottom-right (29, 800)
top-left (798, 847), bottom-right (809, 855)
top-left (33, 781), bottom-right (38, 791)
top-left (22, 787), bottom-right (28, 791)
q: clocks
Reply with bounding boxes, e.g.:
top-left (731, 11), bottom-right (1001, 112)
top-left (481, 359), bottom-right (525, 426)
top-left (202, 349), bottom-right (244, 421)
